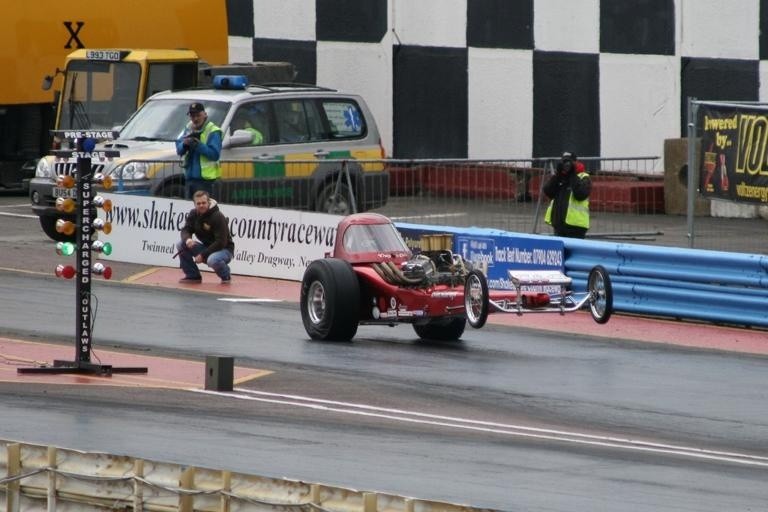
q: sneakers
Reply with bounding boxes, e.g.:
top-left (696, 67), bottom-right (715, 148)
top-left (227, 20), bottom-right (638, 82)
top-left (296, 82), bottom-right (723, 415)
top-left (179, 277), bottom-right (201, 282)
top-left (221, 275), bottom-right (230, 283)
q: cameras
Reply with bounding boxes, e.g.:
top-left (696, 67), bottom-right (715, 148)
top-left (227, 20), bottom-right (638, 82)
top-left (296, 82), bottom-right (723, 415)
top-left (562, 160), bottom-right (574, 173)
top-left (187, 130), bottom-right (201, 144)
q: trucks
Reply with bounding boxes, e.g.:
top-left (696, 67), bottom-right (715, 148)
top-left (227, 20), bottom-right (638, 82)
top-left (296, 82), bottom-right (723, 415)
top-left (30, 46), bottom-right (298, 243)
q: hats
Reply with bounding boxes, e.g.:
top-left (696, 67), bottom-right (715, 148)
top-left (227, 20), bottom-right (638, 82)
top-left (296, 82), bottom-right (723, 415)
top-left (187, 103), bottom-right (204, 115)
top-left (562, 152), bottom-right (576, 161)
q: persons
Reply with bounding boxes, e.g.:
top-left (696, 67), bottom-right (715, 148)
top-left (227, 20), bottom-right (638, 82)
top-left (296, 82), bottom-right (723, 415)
top-left (175, 191), bottom-right (237, 284)
top-left (231, 111), bottom-right (264, 146)
top-left (540, 152), bottom-right (593, 241)
top-left (174, 102), bottom-right (224, 204)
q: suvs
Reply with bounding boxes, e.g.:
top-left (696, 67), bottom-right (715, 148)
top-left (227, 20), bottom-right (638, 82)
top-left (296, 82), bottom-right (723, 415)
top-left (30, 72), bottom-right (389, 246)
